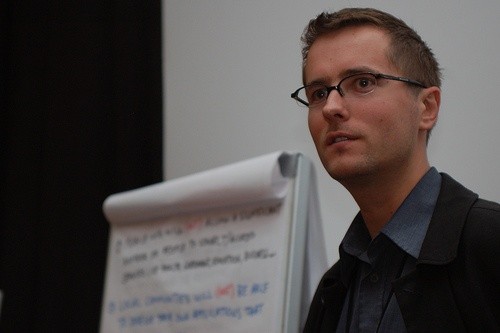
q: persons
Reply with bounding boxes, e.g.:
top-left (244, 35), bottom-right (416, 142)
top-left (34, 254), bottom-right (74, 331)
top-left (290, 7), bottom-right (500, 333)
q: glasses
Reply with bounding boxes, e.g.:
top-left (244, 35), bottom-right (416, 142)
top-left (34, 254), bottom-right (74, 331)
top-left (291, 72), bottom-right (428, 107)
